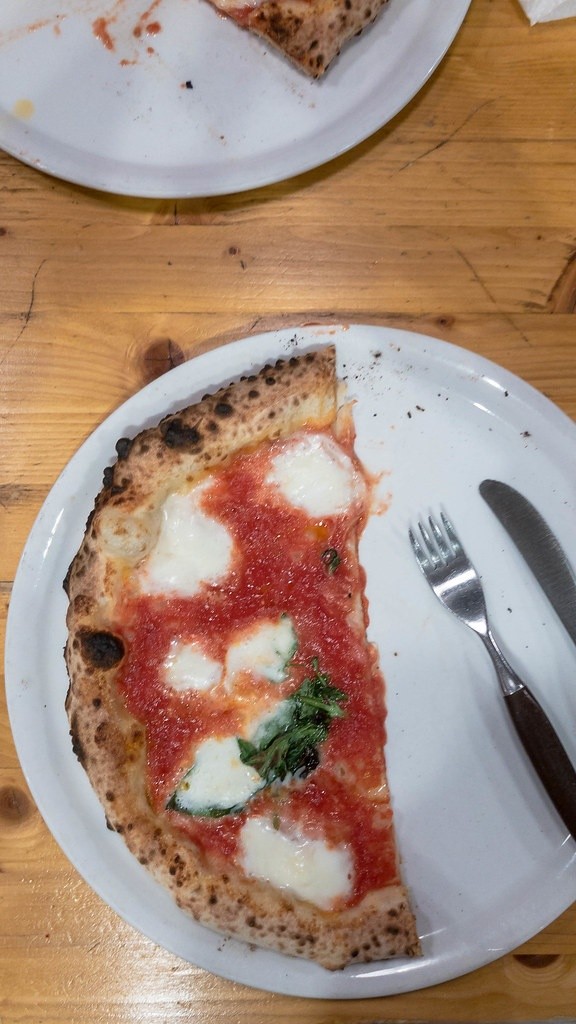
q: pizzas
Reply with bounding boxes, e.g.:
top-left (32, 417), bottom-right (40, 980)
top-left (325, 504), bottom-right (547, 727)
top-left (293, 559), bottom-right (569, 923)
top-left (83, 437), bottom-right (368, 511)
top-left (205, 0), bottom-right (387, 80)
top-left (65, 347), bottom-right (420, 970)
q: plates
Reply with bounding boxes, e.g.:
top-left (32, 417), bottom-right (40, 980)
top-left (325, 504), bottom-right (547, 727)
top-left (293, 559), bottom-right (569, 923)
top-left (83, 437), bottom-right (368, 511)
top-left (0, 0), bottom-right (473, 200)
top-left (4, 323), bottom-right (576, 1001)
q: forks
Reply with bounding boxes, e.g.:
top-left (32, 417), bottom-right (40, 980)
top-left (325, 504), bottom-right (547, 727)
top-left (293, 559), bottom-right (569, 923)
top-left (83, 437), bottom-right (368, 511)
top-left (408, 512), bottom-right (576, 844)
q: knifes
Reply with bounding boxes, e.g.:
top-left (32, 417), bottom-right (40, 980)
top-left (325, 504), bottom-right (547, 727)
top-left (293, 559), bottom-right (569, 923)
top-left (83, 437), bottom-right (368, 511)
top-left (479, 480), bottom-right (575, 648)
